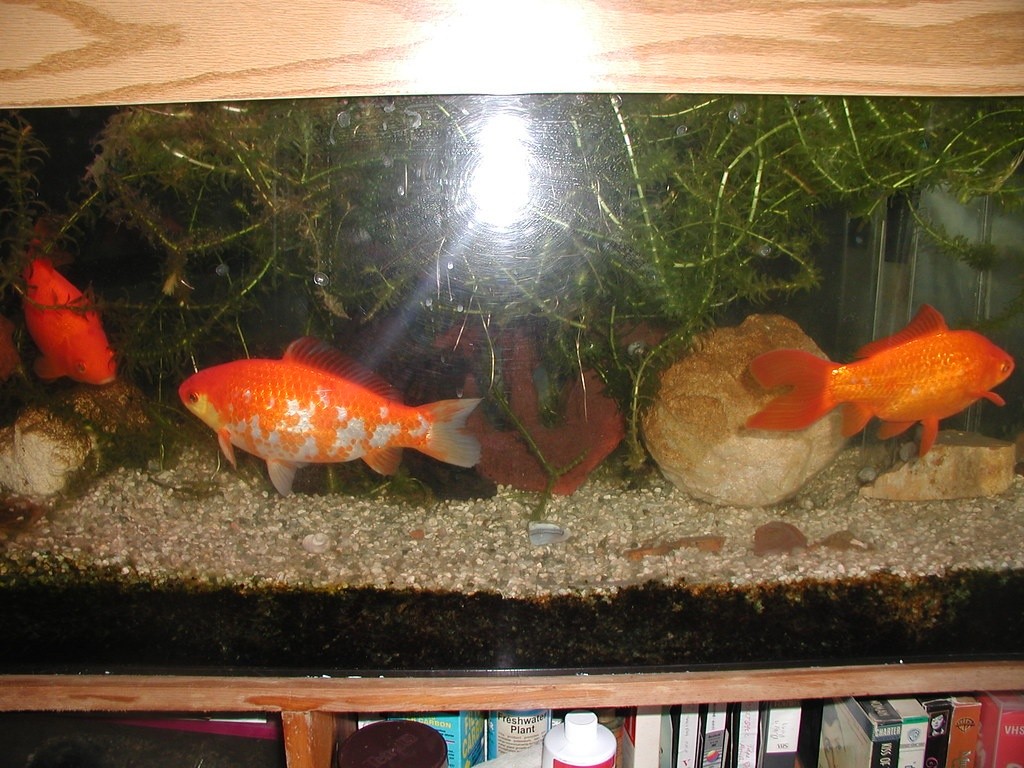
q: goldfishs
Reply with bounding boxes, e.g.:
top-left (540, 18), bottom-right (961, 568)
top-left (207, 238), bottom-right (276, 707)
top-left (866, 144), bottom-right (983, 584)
top-left (1, 221), bottom-right (1015, 498)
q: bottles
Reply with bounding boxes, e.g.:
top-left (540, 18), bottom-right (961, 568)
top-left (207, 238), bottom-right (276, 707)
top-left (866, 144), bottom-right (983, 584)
top-left (594, 707), bottom-right (624, 768)
top-left (543, 709), bottom-right (618, 768)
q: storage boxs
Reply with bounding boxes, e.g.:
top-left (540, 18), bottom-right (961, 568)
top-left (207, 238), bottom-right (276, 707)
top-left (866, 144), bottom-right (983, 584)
top-left (0, 0), bottom-right (1024, 711)
top-left (975, 691), bottom-right (1024, 768)
top-left (386, 711), bottom-right (484, 768)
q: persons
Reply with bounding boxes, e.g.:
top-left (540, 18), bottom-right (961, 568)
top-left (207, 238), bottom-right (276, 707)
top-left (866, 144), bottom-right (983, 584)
top-left (929, 713), bottom-right (946, 735)
top-left (821, 698), bottom-right (847, 768)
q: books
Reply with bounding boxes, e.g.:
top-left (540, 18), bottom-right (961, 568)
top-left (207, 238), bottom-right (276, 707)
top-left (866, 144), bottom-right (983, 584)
top-left (623, 691), bottom-right (1023, 768)
